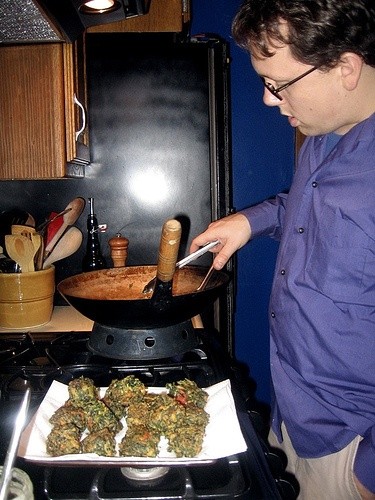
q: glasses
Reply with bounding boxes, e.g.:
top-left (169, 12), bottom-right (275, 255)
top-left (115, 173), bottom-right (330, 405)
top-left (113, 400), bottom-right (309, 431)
top-left (263, 52), bottom-right (340, 101)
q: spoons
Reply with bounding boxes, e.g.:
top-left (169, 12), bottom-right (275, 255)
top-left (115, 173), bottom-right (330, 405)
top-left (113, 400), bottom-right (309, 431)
top-left (41, 195), bottom-right (86, 257)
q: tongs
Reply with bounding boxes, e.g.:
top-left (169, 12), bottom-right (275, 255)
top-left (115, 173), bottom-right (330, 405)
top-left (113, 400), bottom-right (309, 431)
top-left (142, 240), bottom-right (223, 299)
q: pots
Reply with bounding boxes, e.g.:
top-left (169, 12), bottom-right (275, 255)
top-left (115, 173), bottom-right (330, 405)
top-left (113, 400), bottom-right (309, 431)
top-left (57, 267), bottom-right (239, 328)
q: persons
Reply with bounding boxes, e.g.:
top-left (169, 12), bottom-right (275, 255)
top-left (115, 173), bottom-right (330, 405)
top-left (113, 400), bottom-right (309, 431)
top-left (190, 0), bottom-right (375, 499)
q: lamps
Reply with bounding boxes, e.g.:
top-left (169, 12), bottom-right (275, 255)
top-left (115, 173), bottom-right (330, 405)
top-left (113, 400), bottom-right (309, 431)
top-left (77, 0), bottom-right (122, 15)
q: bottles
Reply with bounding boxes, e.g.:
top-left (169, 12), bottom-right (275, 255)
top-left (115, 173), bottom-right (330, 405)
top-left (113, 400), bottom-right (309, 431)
top-left (87, 197), bottom-right (105, 270)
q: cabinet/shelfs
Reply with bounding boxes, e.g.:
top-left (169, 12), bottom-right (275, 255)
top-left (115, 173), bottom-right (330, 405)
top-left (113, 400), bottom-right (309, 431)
top-left (87, 0), bottom-right (191, 32)
top-left (0, 42), bottom-right (92, 180)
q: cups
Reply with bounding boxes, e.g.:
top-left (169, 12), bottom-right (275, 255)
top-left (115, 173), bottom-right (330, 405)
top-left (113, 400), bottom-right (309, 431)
top-left (0, 260), bottom-right (55, 330)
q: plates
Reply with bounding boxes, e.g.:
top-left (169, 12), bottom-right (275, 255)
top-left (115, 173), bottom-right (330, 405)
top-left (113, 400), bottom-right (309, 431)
top-left (17, 379), bottom-right (249, 465)
